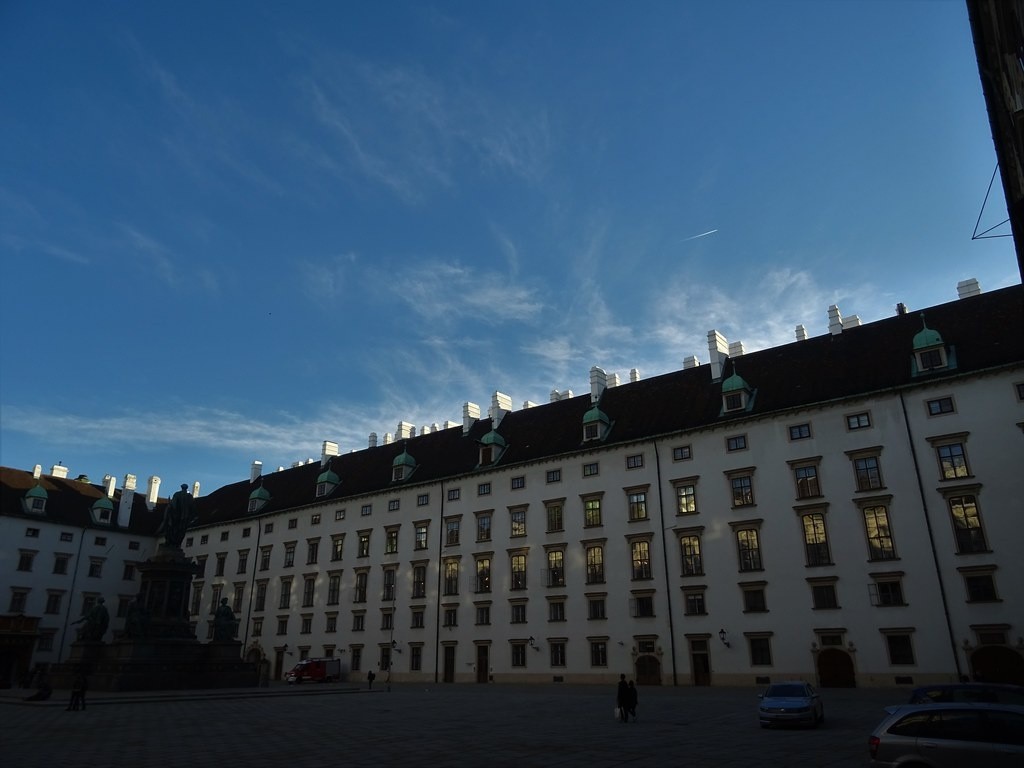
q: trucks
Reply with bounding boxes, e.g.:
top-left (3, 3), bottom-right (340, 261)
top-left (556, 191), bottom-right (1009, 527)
top-left (285, 657), bottom-right (341, 684)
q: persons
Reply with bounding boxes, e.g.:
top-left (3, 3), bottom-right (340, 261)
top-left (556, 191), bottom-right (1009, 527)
top-left (165, 484), bottom-right (193, 548)
top-left (78, 597), bottom-right (110, 641)
top-left (214, 597), bottom-right (236, 641)
top-left (616, 674), bottom-right (638, 722)
top-left (65, 649), bottom-right (93, 711)
top-left (367, 671), bottom-right (373, 690)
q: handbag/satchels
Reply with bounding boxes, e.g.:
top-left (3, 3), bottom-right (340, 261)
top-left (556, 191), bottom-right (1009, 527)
top-left (615, 708), bottom-right (621, 721)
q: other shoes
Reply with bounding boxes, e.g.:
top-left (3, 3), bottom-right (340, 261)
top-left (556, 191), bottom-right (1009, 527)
top-left (632, 716), bottom-right (638, 724)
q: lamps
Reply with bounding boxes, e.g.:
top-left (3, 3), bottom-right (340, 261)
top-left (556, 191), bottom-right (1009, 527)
top-left (283, 643), bottom-right (294, 656)
top-left (391, 640), bottom-right (401, 654)
top-left (617, 641), bottom-right (623, 646)
top-left (719, 628), bottom-right (730, 649)
top-left (528, 636), bottom-right (539, 652)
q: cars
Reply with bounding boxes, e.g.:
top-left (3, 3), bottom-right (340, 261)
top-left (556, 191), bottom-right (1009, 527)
top-left (909, 684), bottom-right (1024, 705)
top-left (758, 680), bottom-right (823, 726)
top-left (868, 705), bottom-right (1024, 768)
